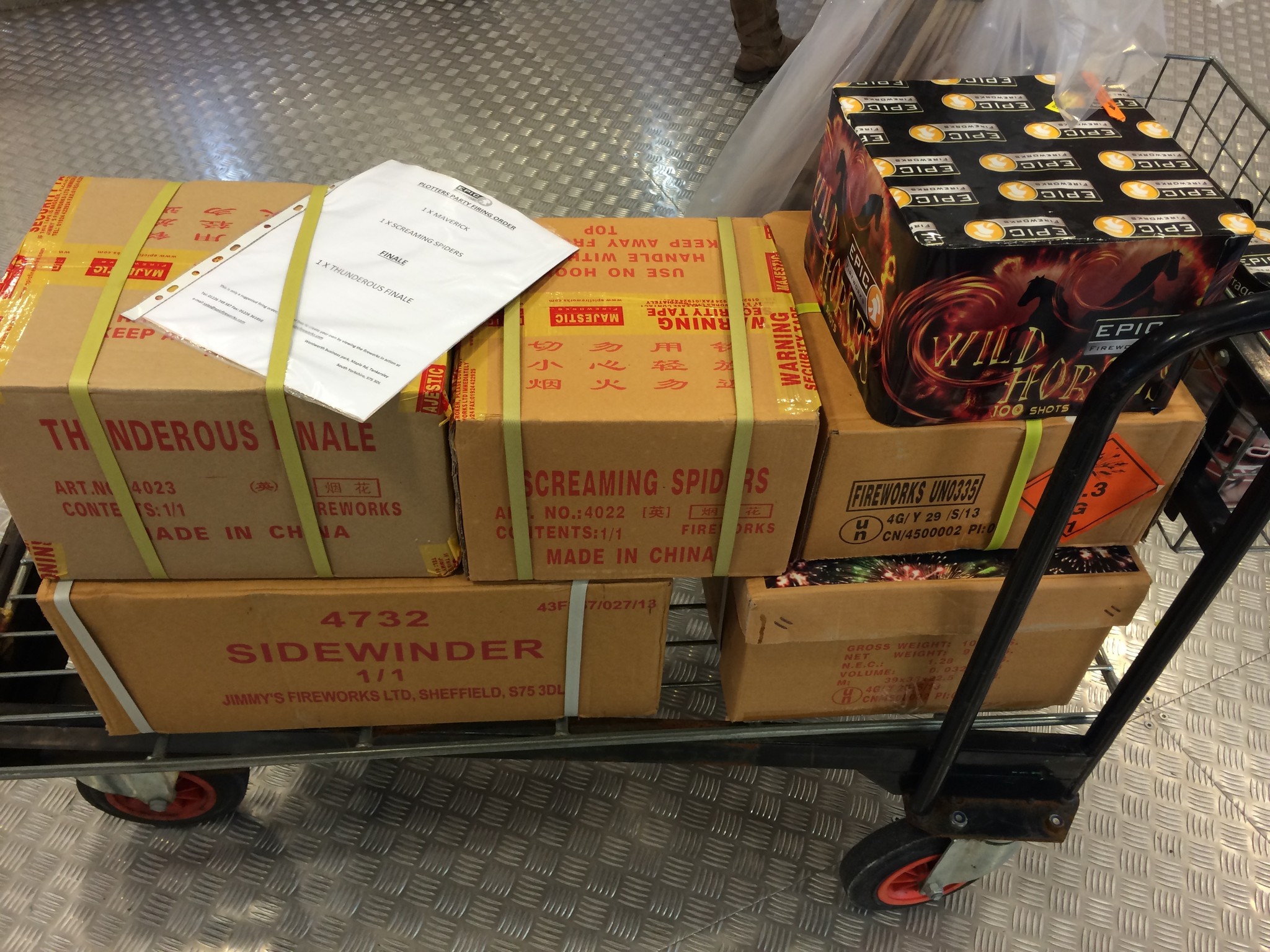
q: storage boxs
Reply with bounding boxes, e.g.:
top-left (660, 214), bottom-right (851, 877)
top-left (0, 66), bottom-right (1263, 743)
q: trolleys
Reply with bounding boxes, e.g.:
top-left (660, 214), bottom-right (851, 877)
top-left (2, 53), bottom-right (1269, 909)
